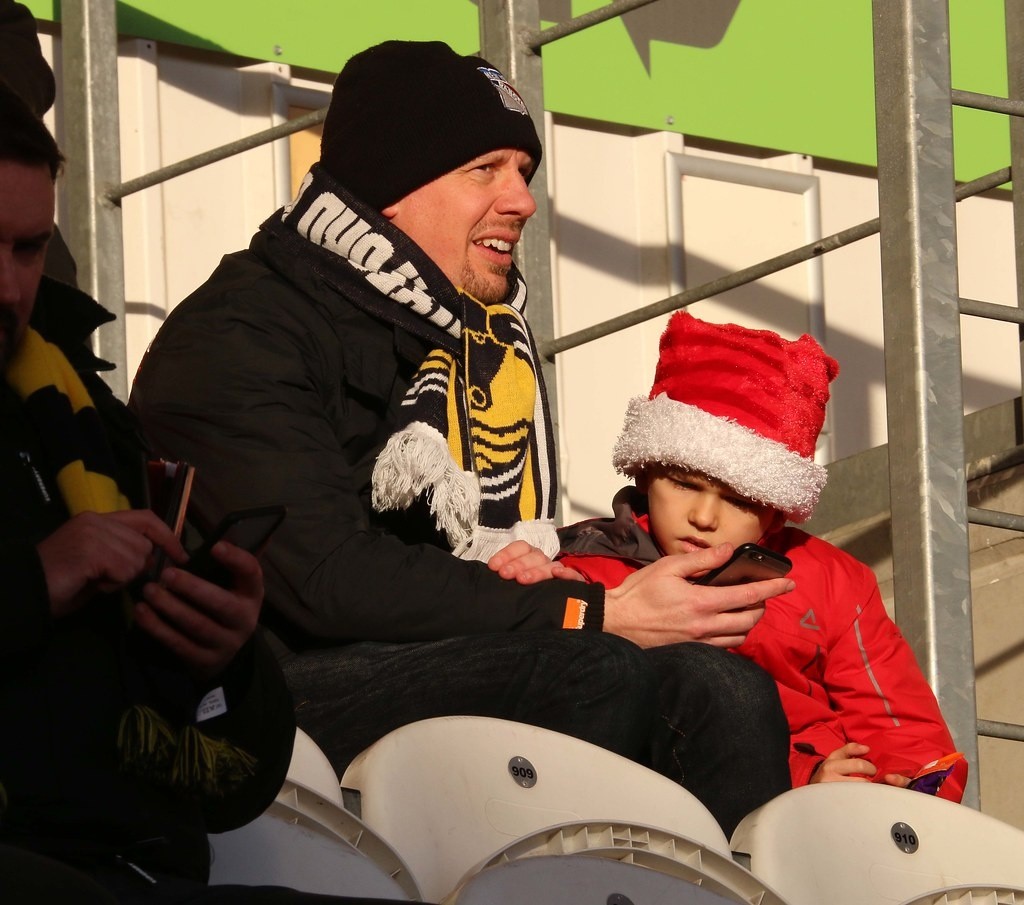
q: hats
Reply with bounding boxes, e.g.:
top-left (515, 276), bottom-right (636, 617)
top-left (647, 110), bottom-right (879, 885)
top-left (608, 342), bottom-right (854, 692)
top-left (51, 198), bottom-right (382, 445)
top-left (320, 41), bottom-right (543, 211)
top-left (613, 310), bottom-right (839, 526)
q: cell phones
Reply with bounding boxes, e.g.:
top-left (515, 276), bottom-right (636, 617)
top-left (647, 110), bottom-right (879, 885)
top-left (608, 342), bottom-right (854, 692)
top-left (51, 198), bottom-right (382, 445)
top-left (167, 505), bottom-right (288, 612)
top-left (690, 542), bottom-right (792, 587)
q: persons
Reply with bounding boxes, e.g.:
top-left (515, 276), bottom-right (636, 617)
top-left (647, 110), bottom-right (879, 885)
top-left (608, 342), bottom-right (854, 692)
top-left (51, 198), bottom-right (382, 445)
top-left (560, 313), bottom-right (970, 807)
top-left (127, 38), bottom-right (793, 834)
top-left (0, 0), bottom-right (300, 902)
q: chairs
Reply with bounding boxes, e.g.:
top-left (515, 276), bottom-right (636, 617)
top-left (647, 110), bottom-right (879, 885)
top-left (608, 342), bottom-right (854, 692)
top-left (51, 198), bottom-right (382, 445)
top-left (207, 716), bottom-right (1024, 905)
